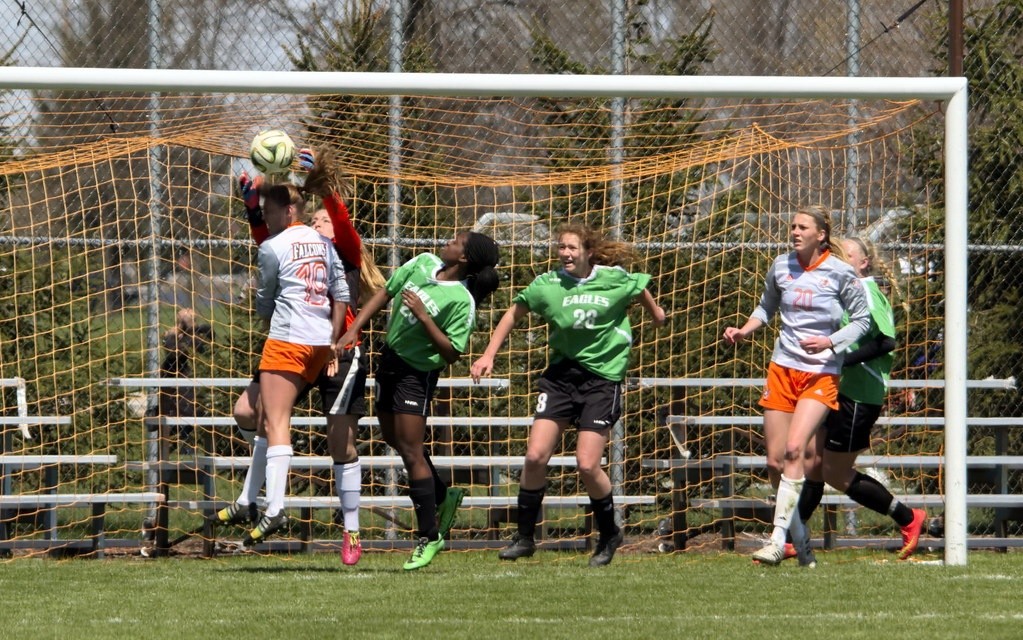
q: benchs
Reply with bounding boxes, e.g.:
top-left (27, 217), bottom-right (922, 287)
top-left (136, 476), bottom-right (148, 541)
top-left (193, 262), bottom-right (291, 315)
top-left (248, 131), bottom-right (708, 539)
top-left (0, 377), bottom-right (1023, 556)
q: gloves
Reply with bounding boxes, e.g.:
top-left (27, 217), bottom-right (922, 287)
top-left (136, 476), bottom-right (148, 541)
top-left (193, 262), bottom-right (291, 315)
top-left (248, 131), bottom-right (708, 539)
top-left (298, 147), bottom-right (317, 174)
top-left (239, 171), bottom-right (265, 220)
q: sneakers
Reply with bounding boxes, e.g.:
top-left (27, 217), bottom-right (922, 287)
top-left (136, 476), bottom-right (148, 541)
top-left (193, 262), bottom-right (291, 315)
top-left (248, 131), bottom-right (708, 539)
top-left (243, 509), bottom-right (289, 546)
top-left (403, 533), bottom-right (446, 571)
top-left (752, 544), bottom-right (785, 564)
top-left (341, 528), bottom-right (362, 565)
top-left (794, 524), bottom-right (816, 569)
top-left (895, 508), bottom-right (925, 559)
top-left (208, 502), bottom-right (260, 527)
top-left (590, 527), bottom-right (623, 567)
top-left (435, 486), bottom-right (464, 536)
top-left (754, 542), bottom-right (797, 564)
top-left (499, 535), bottom-right (537, 559)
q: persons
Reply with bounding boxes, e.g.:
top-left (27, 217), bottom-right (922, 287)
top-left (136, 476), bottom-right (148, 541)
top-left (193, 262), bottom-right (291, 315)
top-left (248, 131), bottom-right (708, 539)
top-left (207, 183), bottom-right (350, 547)
top-left (753, 236), bottom-right (927, 564)
top-left (159, 308), bottom-right (218, 452)
top-left (723, 203), bottom-right (871, 569)
top-left (470, 221), bottom-right (664, 568)
top-left (341, 232), bottom-right (501, 570)
top-left (233, 148), bottom-right (387, 565)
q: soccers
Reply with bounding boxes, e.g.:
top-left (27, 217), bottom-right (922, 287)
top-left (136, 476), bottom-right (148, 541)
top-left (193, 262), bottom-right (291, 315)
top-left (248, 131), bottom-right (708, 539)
top-left (249, 127), bottom-right (296, 173)
